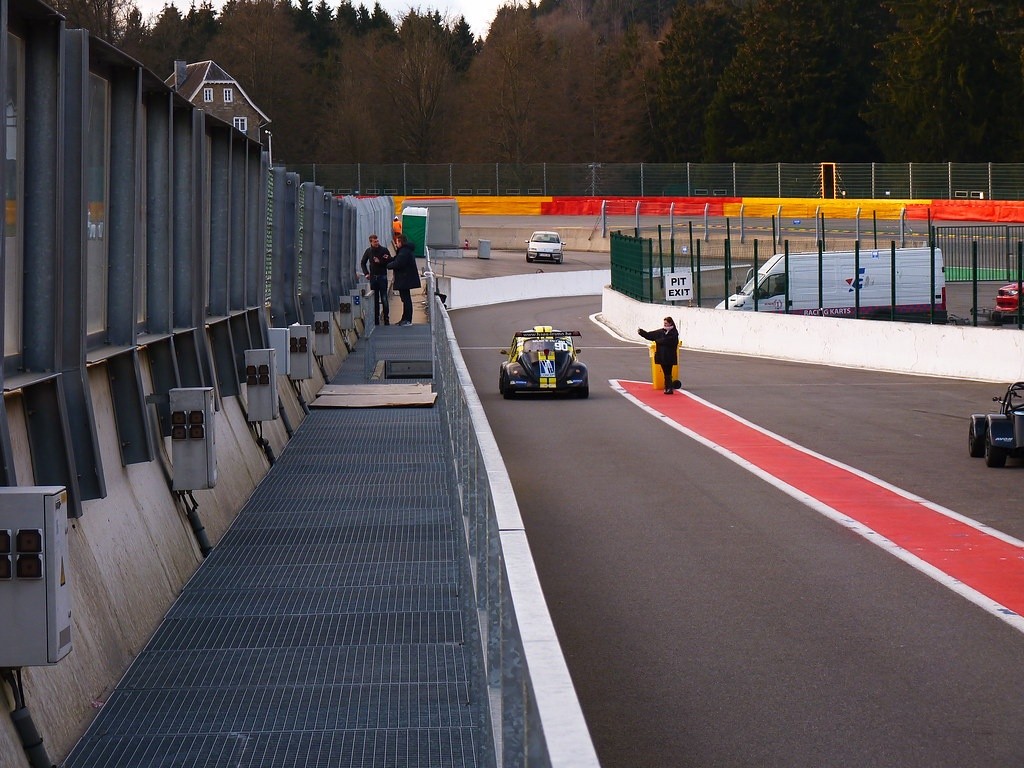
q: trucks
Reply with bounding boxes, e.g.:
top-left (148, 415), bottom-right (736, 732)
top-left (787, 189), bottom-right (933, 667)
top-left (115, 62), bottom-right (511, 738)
top-left (714, 246), bottom-right (947, 324)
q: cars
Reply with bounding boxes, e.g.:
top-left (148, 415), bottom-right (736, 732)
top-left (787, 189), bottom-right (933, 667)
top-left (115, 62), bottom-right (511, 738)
top-left (968, 381), bottom-right (1023, 467)
top-left (996, 282), bottom-right (1024, 312)
top-left (525, 231), bottom-right (566, 264)
top-left (499, 325), bottom-right (589, 400)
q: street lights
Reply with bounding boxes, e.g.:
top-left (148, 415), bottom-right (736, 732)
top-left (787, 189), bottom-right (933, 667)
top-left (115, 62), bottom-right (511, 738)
top-left (264, 130), bottom-right (272, 168)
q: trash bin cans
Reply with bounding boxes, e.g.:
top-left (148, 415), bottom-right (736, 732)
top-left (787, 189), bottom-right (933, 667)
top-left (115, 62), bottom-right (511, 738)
top-left (477, 239), bottom-right (491, 259)
top-left (649, 340), bottom-right (683, 389)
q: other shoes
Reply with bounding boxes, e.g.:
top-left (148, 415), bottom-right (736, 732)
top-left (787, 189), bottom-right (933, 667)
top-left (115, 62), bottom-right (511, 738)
top-left (664, 388), bottom-right (673, 394)
top-left (396, 320), bottom-right (403, 325)
top-left (400, 321), bottom-right (412, 326)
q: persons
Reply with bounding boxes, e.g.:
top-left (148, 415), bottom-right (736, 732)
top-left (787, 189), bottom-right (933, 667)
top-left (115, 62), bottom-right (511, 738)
top-left (768, 274), bottom-right (786, 295)
top-left (374, 234), bottom-right (421, 327)
top-left (361, 235), bottom-right (392, 327)
top-left (464, 239), bottom-right (471, 250)
top-left (637, 317), bottom-right (681, 394)
top-left (392, 215), bottom-right (403, 235)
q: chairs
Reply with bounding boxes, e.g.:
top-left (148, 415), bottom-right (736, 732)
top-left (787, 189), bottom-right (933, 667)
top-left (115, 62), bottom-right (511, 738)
top-left (550, 237), bottom-right (555, 241)
